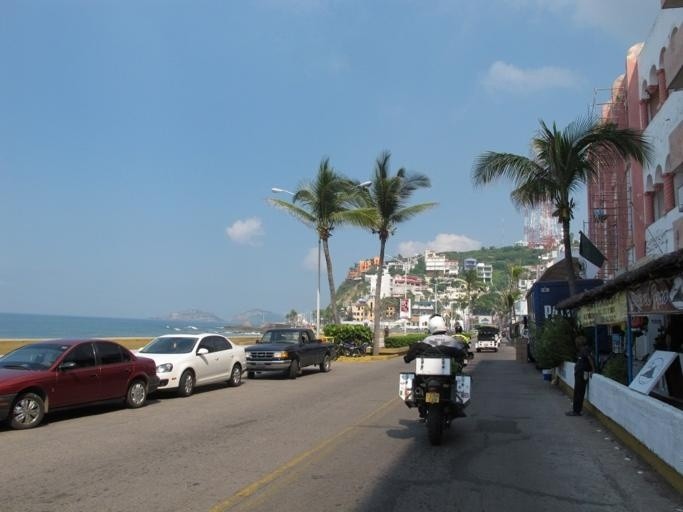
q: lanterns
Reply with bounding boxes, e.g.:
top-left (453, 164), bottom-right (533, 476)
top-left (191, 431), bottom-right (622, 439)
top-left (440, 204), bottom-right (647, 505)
top-left (631, 317), bottom-right (644, 331)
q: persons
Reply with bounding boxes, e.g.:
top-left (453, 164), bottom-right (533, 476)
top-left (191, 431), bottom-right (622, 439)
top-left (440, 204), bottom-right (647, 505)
top-left (653, 325), bottom-right (669, 350)
top-left (451, 325), bottom-right (472, 365)
top-left (564, 335), bottom-right (596, 417)
top-left (403, 315), bottom-right (465, 424)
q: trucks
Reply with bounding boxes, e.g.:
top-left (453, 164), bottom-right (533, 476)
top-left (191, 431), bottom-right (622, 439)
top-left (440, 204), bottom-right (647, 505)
top-left (524, 277), bottom-right (609, 365)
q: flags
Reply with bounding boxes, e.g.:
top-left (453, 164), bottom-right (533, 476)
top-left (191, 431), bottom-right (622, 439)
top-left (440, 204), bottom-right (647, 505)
top-left (580, 232), bottom-right (605, 268)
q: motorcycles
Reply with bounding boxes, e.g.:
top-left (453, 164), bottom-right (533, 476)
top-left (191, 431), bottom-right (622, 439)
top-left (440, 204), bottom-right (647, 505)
top-left (333, 340), bottom-right (368, 359)
top-left (398, 335), bottom-right (472, 446)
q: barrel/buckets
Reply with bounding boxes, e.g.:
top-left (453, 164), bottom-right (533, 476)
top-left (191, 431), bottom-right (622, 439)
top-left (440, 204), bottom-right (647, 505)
top-left (542, 368), bottom-right (553, 381)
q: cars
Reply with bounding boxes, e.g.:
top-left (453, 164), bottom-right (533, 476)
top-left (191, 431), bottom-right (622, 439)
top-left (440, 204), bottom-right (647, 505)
top-left (473, 322), bottom-right (502, 354)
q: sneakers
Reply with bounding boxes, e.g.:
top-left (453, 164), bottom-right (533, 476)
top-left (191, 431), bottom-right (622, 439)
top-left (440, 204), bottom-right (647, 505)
top-left (566, 409), bottom-right (584, 416)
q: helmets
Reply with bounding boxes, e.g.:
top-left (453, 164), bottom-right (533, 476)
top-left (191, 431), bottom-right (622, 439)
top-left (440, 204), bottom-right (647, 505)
top-left (428, 314), bottom-right (447, 335)
top-left (455, 325), bottom-right (463, 333)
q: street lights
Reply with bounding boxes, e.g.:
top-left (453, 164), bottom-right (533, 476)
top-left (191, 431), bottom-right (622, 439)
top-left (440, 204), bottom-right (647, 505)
top-left (383, 250), bottom-right (436, 334)
top-left (270, 181), bottom-right (373, 339)
